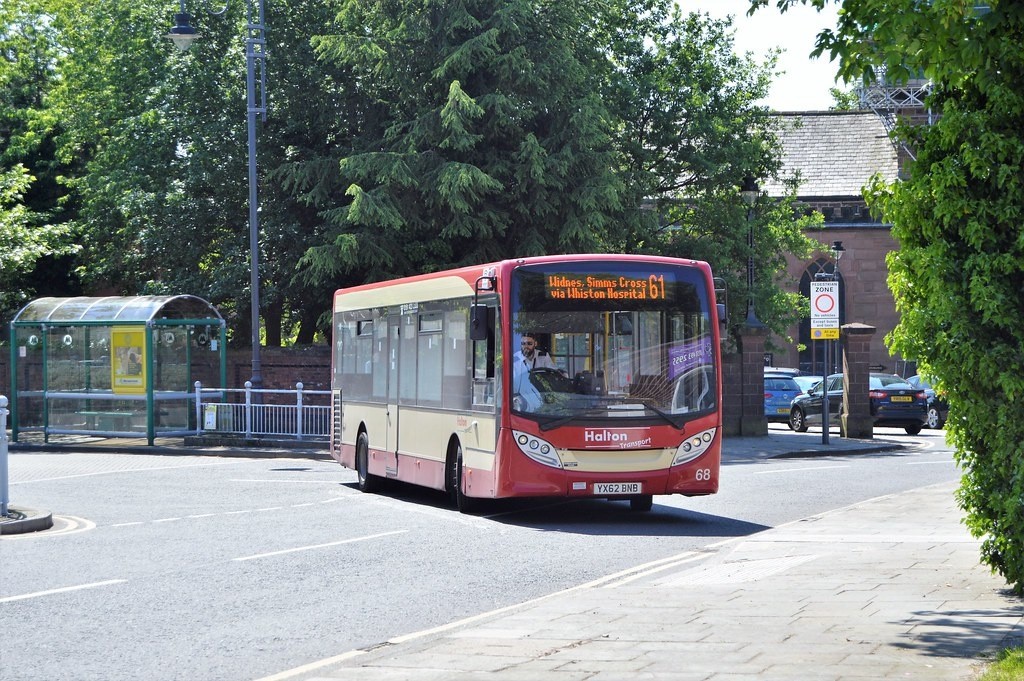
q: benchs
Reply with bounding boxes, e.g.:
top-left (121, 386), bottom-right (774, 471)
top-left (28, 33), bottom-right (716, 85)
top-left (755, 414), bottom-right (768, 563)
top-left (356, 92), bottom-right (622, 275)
top-left (78, 411), bottom-right (168, 432)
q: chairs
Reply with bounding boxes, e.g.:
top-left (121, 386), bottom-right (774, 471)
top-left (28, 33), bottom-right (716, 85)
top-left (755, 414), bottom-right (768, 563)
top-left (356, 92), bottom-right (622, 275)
top-left (573, 370), bottom-right (608, 417)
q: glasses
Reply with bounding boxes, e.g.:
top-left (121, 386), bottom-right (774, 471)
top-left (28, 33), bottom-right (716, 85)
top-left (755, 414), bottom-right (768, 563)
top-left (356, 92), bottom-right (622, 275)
top-left (521, 342), bottom-right (533, 345)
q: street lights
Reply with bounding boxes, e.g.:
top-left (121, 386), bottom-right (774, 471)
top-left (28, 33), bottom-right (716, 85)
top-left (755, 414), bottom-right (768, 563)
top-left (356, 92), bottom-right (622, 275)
top-left (812, 240), bottom-right (848, 444)
top-left (169, 0), bottom-right (266, 403)
top-left (738, 168), bottom-right (769, 323)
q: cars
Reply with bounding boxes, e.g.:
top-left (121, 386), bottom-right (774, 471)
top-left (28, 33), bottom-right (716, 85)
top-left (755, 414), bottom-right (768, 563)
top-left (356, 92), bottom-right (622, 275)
top-left (764, 366), bottom-right (823, 395)
top-left (788, 371), bottom-right (929, 436)
top-left (902, 373), bottom-right (957, 430)
top-left (763, 374), bottom-right (805, 430)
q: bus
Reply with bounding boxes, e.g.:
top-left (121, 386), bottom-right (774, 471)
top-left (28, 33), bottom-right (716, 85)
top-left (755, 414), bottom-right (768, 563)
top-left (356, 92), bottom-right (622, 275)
top-left (330, 254), bottom-right (730, 513)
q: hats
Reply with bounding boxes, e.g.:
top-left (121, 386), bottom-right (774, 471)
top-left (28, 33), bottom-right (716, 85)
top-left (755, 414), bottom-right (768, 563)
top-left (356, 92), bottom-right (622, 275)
top-left (521, 331), bottom-right (536, 338)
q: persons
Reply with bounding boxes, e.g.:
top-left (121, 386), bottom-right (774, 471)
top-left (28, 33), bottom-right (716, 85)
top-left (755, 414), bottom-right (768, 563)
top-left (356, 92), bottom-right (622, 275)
top-left (364, 349), bottom-right (384, 373)
top-left (512, 332), bottom-right (567, 410)
